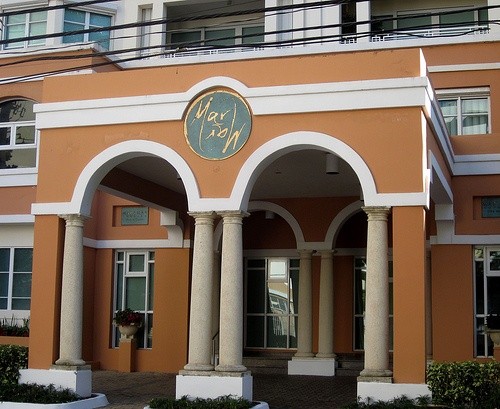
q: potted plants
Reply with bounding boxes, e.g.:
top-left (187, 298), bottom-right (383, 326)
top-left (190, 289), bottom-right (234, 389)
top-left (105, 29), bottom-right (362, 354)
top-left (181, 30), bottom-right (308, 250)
top-left (112, 308), bottom-right (143, 341)
